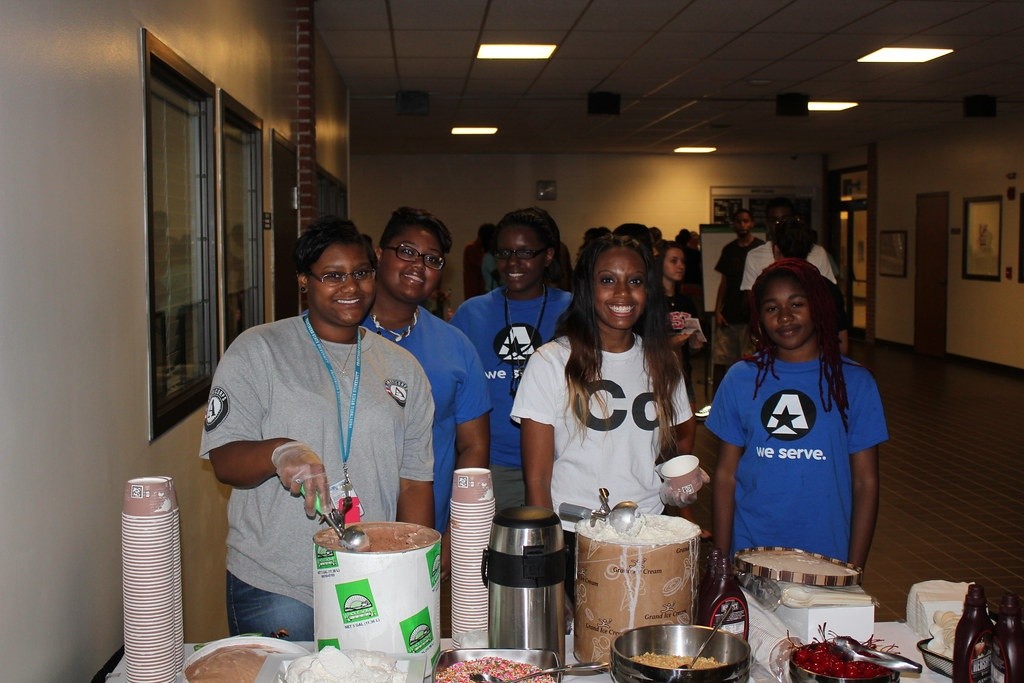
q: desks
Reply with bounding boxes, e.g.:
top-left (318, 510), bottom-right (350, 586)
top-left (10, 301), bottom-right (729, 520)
top-left (95, 641), bottom-right (956, 683)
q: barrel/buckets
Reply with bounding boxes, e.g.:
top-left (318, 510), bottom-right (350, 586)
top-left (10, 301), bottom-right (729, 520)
top-left (610, 624), bottom-right (751, 683)
top-left (574, 515), bottom-right (702, 672)
top-left (789, 646), bottom-right (900, 683)
top-left (312, 522), bottom-right (442, 683)
top-left (432, 648), bottom-right (562, 683)
top-left (183, 637), bottom-right (312, 683)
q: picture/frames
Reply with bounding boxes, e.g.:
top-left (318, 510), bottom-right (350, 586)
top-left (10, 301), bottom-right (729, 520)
top-left (961, 193), bottom-right (1003, 281)
top-left (879, 231), bottom-right (908, 277)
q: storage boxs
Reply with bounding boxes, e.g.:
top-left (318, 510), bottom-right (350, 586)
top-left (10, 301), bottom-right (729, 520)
top-left (772, 602), bottom-right (874, 645)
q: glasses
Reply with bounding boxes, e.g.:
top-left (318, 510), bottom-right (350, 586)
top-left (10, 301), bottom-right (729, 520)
top-left (308, 266), bottom-right (376, 285)
top-left (494, 246), bottom-right (550, 260)
top-left (385, 245), bottom-right (445, 271)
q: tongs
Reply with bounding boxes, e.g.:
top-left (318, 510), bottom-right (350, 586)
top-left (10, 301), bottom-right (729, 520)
top-left (830, 636), bottom-right (922, 674)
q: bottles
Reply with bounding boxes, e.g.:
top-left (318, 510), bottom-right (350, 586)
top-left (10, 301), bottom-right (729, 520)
top-left (700, 549), bottom-right (749, 641)
top-left (953, 585), bottom-right (1024, 683)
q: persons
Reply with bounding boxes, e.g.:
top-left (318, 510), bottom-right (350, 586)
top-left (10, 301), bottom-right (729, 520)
top-left (445, 206), bottom-right (575, 515)
top-left (507, 232), bottom-right (710, 609)
top-left (461, 221), bottom-right (498, 300)
top-left (582, 219), bottom-right (706, 374)
top-left (198, 210), bottom-right (438, 640)
top-left (705, 257), bottom-right (889, 583)
top-left (357, 205), bottom-right (497, 580)
top-left (692, 196), bottom-right (851, 414)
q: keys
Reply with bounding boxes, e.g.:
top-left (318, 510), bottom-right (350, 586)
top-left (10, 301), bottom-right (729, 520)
top-left (342, 482), bottom-right (353, 497)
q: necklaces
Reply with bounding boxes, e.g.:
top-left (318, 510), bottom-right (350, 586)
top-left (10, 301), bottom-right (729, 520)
top-left (369, 305), bottom-right (419, 343)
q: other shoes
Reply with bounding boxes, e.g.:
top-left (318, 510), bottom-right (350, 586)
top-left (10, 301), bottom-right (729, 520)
top-left (693, 404), bottom-right (713, 417)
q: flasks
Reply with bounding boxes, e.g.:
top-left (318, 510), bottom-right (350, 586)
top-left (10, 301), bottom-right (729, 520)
top-left (481, 506), bottom-right (565, 677)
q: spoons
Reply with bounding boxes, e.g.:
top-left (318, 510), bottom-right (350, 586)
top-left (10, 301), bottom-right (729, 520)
top-left (926, 610), bottom-right (963, 658)
top-left (782, 586), bottom-right (872, 608)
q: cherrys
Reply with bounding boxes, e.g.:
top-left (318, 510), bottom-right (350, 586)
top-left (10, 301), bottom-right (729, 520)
top-left (786, 622), bottom-right (902, 678)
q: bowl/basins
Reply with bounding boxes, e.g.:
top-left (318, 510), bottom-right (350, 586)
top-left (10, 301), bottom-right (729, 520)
top-left (450, 468), bottom-right (495, 648)
top-left (660, 455), bottom-right (702, 495)
top-left (122, 475), bottom-right (184, 683)
